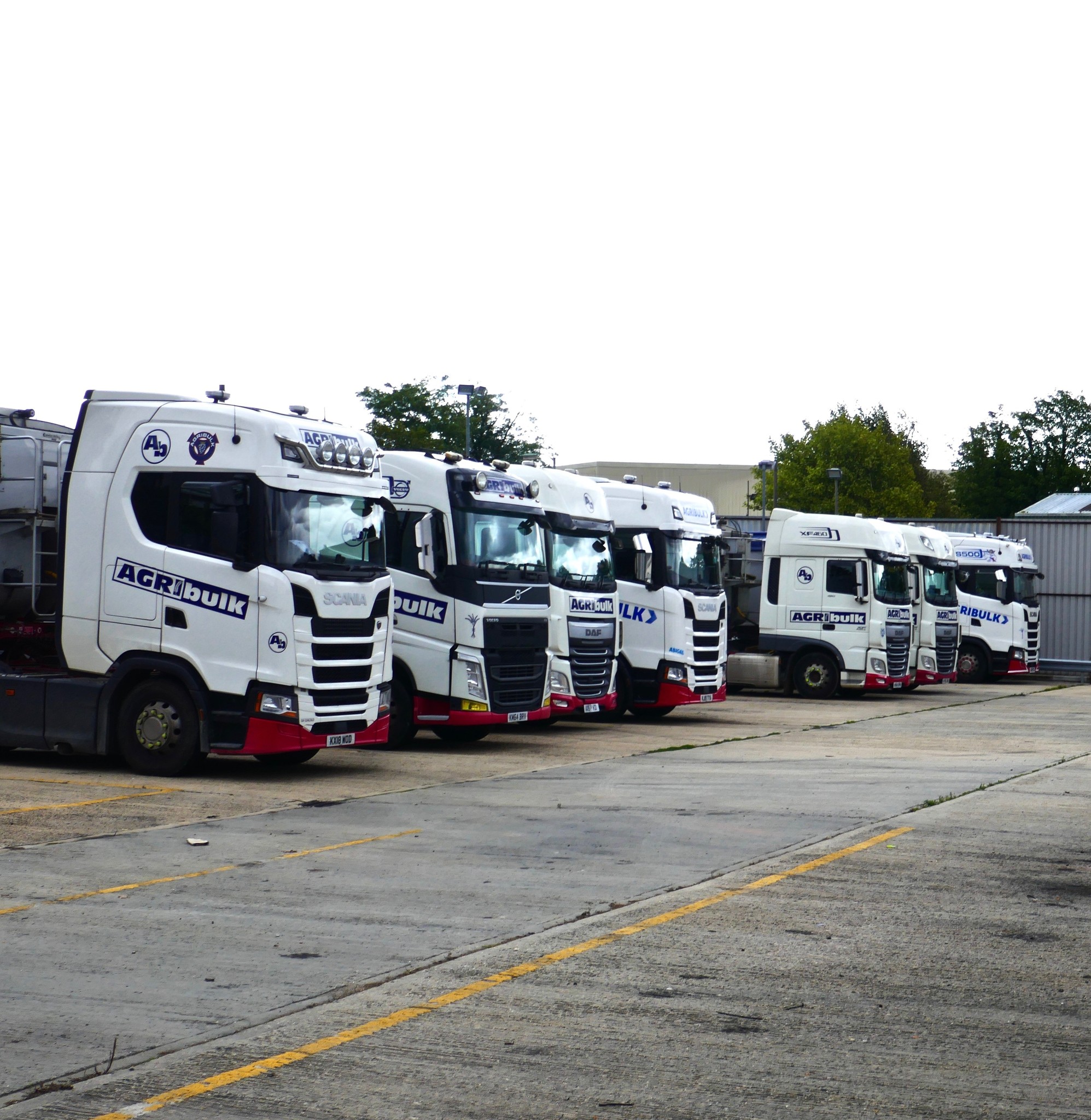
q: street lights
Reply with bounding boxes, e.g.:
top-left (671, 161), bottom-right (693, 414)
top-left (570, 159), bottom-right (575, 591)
top-left (458, 384), bottom-right (486, 457)
top-left (757, 459), bottom-right (774, 532)
top-left (825, 468), bottom-right (842, 515)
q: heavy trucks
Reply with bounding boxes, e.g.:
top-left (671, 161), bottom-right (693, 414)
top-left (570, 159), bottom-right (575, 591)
top-left (0, 378), bottom-right (730, 779)
top-left (715, 506), bottom-right (1046, 700)
